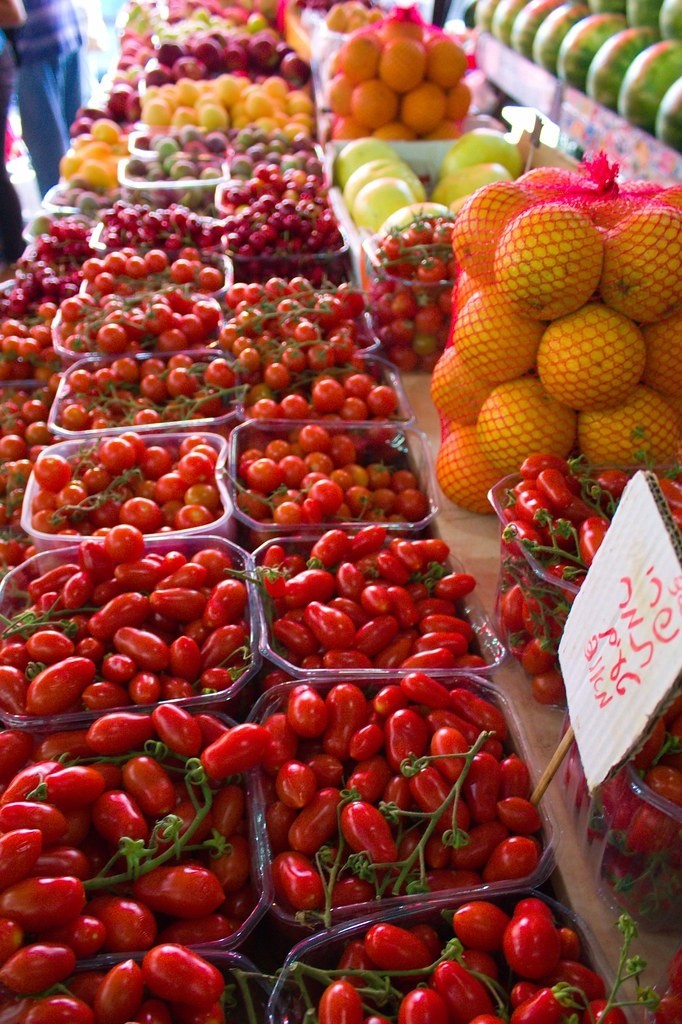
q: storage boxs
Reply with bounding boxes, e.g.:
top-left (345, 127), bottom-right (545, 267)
top-left (0, 31), bottom-right (681, 1023)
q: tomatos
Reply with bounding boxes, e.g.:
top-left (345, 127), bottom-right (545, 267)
top-left (1, 208), bottom-right (682, 1024)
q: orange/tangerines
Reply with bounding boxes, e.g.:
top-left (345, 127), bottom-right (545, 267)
top-left (329, 10), bottom-right (471, 142)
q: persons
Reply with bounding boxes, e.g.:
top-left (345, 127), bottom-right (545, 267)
top-left (0, 0), bottom-right (130, 284)
